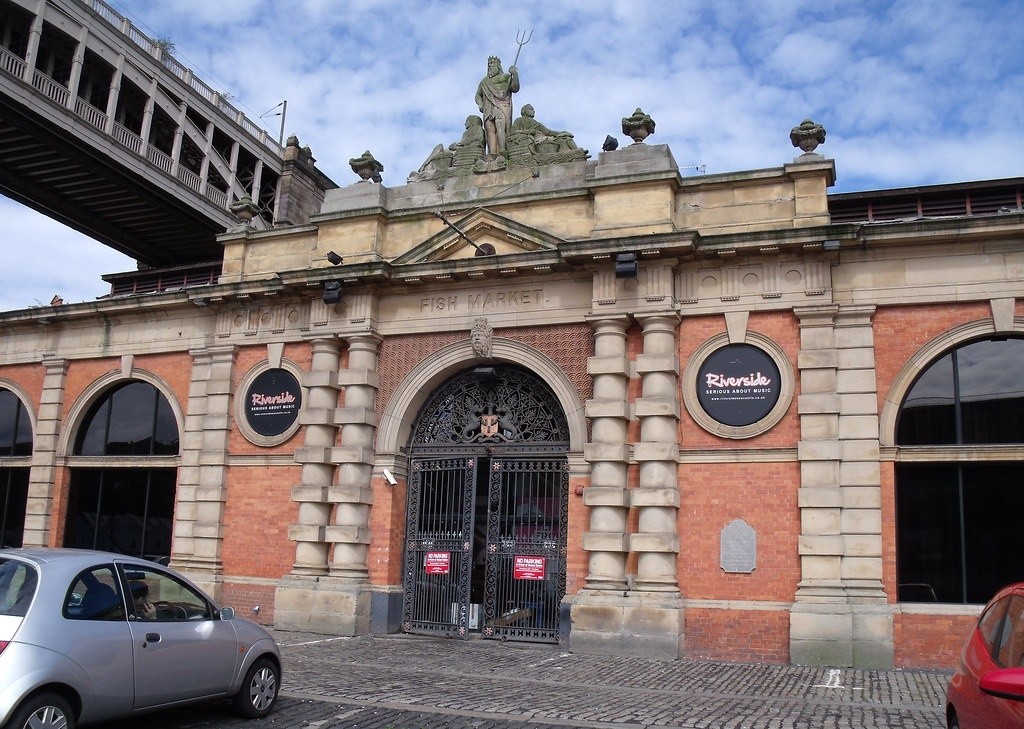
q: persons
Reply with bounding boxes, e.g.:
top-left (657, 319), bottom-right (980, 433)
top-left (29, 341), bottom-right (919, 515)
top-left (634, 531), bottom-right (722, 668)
top-left (416, 55), bottom-right (591, 171)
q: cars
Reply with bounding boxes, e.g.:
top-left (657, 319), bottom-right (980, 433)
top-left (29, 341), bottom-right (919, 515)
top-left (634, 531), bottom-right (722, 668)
top-left (946, 580), bottom-right (1024, 729)
top-left (0, 545), bottom-right (281, 729)
